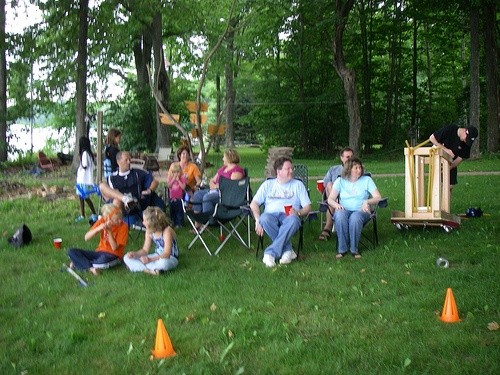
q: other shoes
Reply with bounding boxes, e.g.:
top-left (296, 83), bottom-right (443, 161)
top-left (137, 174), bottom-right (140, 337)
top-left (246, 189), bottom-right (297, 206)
top-left (91, 214), bottom-right (98, 222)
top-left (262, 253), bottom-right (276, 268)
top-left (279, 249), bottom-right (297, 264)
top-left (75, 216), bottom-right (84, 223)
top-left (335, 251), bottom-right (347, 259)
top-left (351, 251), bottom-right (362, 259)
top-left (188, 227), bottom-right (206, 234)
top-left (124, 197), bottom-right (138, 212)
top-left (154, 268), bottom-right (164, 277)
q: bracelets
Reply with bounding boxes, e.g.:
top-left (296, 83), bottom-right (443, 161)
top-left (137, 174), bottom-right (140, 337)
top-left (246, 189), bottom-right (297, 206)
top-left (255, 221), bottom-right (260, 223)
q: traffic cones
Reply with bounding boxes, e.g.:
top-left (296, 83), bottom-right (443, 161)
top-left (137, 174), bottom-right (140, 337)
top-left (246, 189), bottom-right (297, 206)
top-left (438, 287), bottom-right (462, 322)
top-left (151, 319), bottom-right (177, 358)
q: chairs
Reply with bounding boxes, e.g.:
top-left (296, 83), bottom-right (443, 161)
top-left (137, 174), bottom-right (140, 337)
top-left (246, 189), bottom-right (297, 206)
top-left (163, 163), bottom-right (254, 256)
top-left (39, 152), bottom-right (60, 171)
top-left (316, 173), bottom-right (389, 250)
top-left (97, 158), bottom-right (164, 241)
top-left (256, 165), bottom-right (320, 261)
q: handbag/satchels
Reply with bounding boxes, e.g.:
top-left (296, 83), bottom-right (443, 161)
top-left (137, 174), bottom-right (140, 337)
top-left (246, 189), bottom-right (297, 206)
top-left (465, 207), bottom-right (483, 219)
top-left (133, 168), bottom-right (156, 210)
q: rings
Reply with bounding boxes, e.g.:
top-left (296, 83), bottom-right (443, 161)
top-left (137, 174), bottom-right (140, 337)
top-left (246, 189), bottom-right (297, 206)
top-left (367, 209), bottom-right (369, 211)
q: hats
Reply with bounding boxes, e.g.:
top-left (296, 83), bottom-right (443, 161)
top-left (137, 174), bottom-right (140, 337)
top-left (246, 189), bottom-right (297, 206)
top-left (465, 125), bottom-right (478, 147)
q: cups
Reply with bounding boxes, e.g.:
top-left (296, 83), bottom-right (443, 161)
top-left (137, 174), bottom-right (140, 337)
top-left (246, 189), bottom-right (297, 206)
top-left (284, 204), bottom-right (292, 215)
top-left (54, 238), bottom-right (63, 248)
top-left (316, 180), bottom-right (324, 191)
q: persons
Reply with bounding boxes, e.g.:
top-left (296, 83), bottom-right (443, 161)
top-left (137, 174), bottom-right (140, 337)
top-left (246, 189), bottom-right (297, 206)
top-left (123, 206), bottom-right (179, 277)
top-left (167, 164), bottom-right (186, 229)
top-left (428, 123), bottom-right (478, 192)
top-left (100, 151), bottom-right (159, 217)
top-left (168, 146), bottom-right (201, 192)
top-left (104, 128), bottom-right (122, 187)
top-left (318, 146), bottom-right (355, 241)
top-left (75, 136), bottom-right (98, 223)
top-left (67, 203), bottom-right (128, 277)
top-left (249, 157), bottom-right (312, 268)
top-left (189, 148), bottom-right (245, 234)
top-left (328, 157), bottom-right (382, 259)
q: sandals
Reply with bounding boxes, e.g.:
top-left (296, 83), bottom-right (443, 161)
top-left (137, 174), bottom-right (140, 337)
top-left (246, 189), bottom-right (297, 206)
top-left (319, 229), bottom-right (331, 241)
